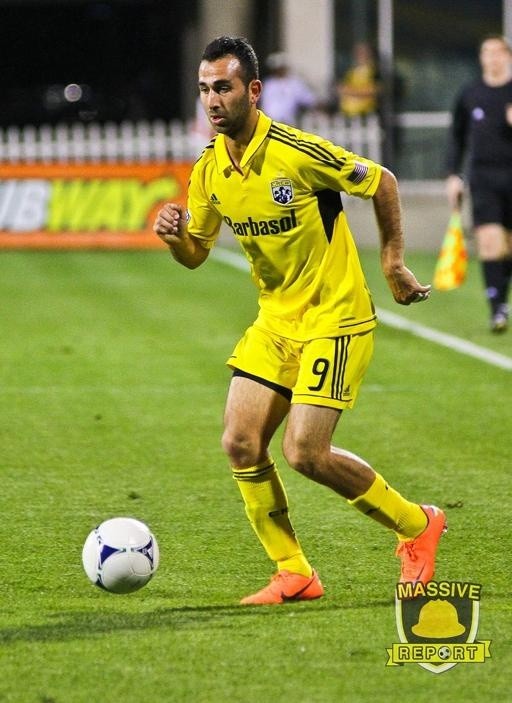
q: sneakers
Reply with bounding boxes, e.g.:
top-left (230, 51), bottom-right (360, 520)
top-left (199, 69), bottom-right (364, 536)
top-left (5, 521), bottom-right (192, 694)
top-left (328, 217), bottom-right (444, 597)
top-left (239, 568), bottom-right (324, 604)
top-left (492, 303), bottom-right (509, 331)
top-left (397, 504), bottom-right (446, 588)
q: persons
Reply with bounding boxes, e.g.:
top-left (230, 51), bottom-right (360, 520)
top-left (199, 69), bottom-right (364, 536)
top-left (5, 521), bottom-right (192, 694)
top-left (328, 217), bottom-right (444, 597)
top-left (443, 37), bottom-right (511, 333)
top-left (151, 36), bottom-right (445, 606)
top-left (333, 41), bottom-right (381, 117)
top-left (257, 51), bottom-right (328, 133)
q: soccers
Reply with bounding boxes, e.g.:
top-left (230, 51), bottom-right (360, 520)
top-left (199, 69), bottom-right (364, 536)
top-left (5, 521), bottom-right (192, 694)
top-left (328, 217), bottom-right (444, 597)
top-left (82, 517), bottom-right (158, 594)
